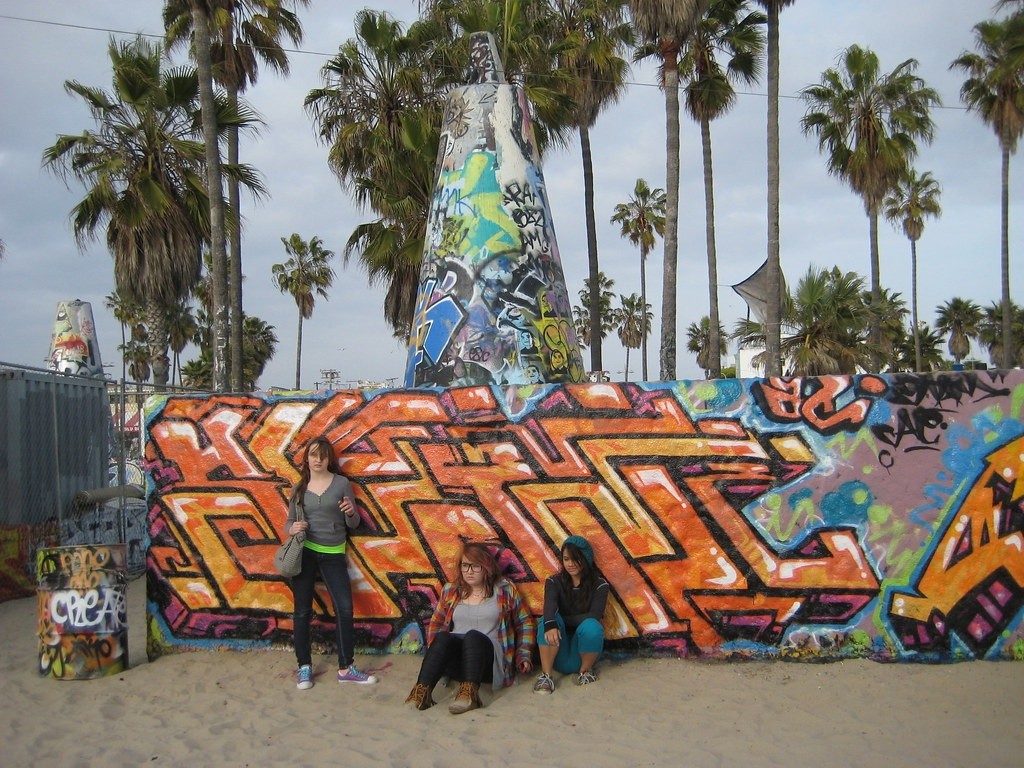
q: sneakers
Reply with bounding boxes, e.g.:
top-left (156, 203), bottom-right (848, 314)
top-left (533, 673), bottom-right (555, 695)
top-left (337, 665), bottom-right (378, 685)
top-left (449, 682), bottom-right (479, 714)
top-left (404, 683), bottom-right (433, 711)
top-left (577, 671), bottom-right (597, 687)
top-left (296, 668), bottom-right (313, 689)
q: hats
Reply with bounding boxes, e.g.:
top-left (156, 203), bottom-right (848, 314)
top-left (564, 535), bottom-right (594, 566)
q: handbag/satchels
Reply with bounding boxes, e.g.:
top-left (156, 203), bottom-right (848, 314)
top-left (273, 527), bottom-right (305, 577)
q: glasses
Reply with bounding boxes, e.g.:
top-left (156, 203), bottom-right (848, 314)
top-left (459, 562), bottom-right (483, 573)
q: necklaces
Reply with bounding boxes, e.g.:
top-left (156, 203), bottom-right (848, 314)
top-left (467, 588), bottom-right (484, 631)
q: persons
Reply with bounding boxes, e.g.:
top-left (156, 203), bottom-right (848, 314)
top-left (534, 535), bottom-right (610, 697)
top-left (403, 542), bottom-right (536, 714)
top-left (283, 435), bottom-right (379, 691)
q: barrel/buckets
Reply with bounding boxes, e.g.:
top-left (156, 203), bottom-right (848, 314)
top-left (34, 542), bottom-right (128, 682)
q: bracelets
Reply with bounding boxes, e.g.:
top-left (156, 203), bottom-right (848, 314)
top-left (347, 512), bottom-right (355, 517)
